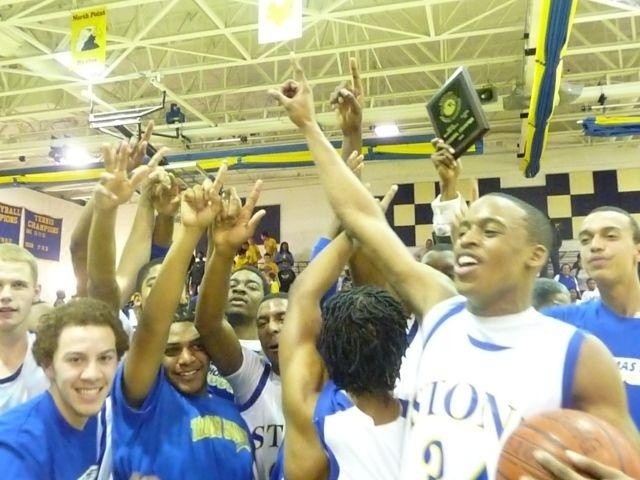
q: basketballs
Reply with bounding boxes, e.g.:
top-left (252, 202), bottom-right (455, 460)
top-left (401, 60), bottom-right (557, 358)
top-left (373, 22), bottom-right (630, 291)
top-left (496, 408), bottom-right (640, 480)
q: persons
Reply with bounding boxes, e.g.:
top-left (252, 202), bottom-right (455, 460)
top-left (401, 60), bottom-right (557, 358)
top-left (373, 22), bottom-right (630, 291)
top-left (277, 183), bottom-right (412, 479)
top-left (1, 243), bottom-right (53, 418)
top-left (537, 207), bottom-right (640, 435)
top-left (28, 53), bottom-right (600, 391)
top-left (109, 161), bottom-right (256, 479)
top-left (268, 49), bottom-right (640, 480)
top-left (192, 176), bottom-right (286, 479)
top-left (0, 295), bottom-right (130, 480)
